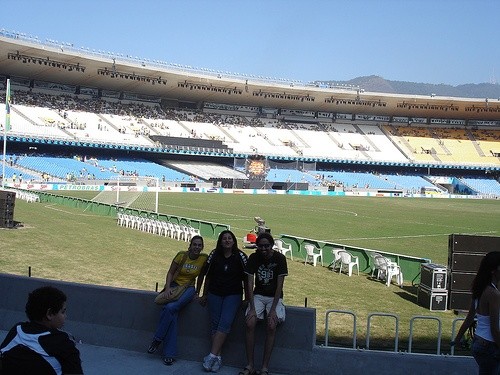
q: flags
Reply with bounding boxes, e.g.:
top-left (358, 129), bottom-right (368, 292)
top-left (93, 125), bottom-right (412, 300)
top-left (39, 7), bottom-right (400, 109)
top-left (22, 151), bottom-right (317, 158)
top-left (4, 79), bottom-right (10, 136)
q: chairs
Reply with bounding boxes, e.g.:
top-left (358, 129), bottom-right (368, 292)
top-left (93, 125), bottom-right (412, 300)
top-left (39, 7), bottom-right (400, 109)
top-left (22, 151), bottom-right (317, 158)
top-left (331, 249), bottom-right (360, 277)
top-left (304, 244), bottom-right (323, 267)
top-left (272, 239), bottom-right (292, 260)
top-left (0, 187), bottom-right (40, 203)
top-left (117, 212), bottom-right (201, 242)
top-left (370, 252), bottom-right (404, 289)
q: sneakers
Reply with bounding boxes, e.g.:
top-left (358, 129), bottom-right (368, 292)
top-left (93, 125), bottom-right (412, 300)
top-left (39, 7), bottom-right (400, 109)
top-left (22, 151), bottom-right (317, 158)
top-left (203, 352), bottom-right (218, 372)
top-left (211, 354), bottom-right (223, 372)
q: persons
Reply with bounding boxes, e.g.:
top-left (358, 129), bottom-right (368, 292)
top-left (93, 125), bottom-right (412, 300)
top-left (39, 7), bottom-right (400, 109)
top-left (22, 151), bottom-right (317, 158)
top-left (200, 230), bottom-right (250, 371)
top-left (266, 164), bottom-right (369, 188)
top-left (144, 175), bottom-right (199, 183)
top-left (89, 156), bottom-right (138, 176)
top-left (0, 86), bottom-right (500, 157)
top-left (238, 232), bottom-right (288, 375)
top-left (370, 168), bottom-right (420, 194)
top-left (0, 148), bottom-right (96, 183)
top-left (147, 235), bottom-right (208, 366)
top-left (0, 284), bottom-right (84, 375)
top-left (452, 249), bottom-right (500, 375)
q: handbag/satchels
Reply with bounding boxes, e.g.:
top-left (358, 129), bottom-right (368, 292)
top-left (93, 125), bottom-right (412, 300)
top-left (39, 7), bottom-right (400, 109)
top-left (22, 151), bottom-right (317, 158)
top-left (155, 286), bottom-right (181, 304)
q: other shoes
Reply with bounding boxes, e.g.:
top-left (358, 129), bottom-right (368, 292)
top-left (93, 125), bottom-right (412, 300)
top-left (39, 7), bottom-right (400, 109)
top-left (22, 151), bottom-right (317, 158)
top-left (238, 365), bottom-right (256, 375)
top-left (163, 357), bottom-right (176, 366)
top-left (260, 368), bottom-right (269, 375)
top-left (147, 339), bottom-right (162, 354)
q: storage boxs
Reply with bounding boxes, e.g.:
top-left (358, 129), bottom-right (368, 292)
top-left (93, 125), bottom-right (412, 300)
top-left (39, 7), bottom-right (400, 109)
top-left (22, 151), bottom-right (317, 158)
top-left (418, 263), bottom-right (449, 312)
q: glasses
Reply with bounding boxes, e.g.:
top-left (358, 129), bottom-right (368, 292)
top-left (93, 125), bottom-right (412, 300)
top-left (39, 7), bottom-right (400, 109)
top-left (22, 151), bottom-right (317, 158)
top-left (258, 243), bottom-right (271, 248)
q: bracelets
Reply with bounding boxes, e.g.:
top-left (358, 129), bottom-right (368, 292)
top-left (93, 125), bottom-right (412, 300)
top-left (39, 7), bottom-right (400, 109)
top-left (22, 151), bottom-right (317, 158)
top-left (195, 291), bottom-right (199, 295)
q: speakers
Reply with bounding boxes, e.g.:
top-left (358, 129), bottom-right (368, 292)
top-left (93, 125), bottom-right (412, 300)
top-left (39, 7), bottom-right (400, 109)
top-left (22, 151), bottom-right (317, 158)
top-left (448, 234), bottom-right (500, 308)
top-left (0, 190), bottom-right (15, 227)
